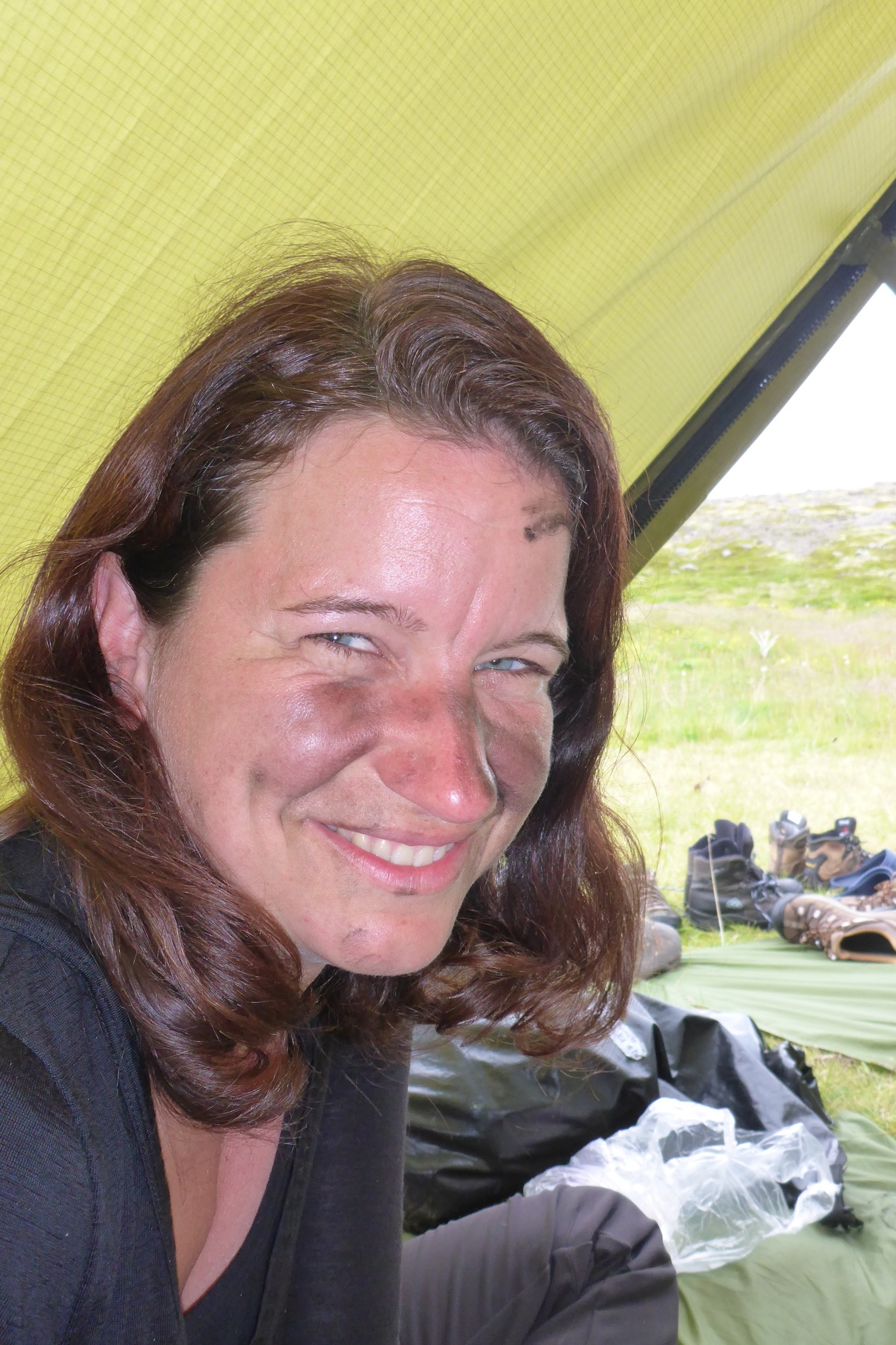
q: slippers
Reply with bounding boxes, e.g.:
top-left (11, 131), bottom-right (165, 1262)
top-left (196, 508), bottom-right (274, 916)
top-left (830, 849), bottom-right (896, 896)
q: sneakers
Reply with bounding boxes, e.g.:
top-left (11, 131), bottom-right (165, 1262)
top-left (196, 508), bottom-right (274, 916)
top-left (635, 918), bottom-right (681, 980)
top-left (804, 816), bottom-right (871, 892)
top-left (687, 822), bottom-right (802, 932)
top-left (680, 820), bottom-right (738, 917)
top-left (767, 810), bottom-right (811, 889)
top-left (771, 893), bottom-right (896, 964)
top-left (626, 862), bottom-right (682, 927)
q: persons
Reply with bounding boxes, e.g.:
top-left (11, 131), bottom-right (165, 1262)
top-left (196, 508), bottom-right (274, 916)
top-left (0, 251), bottom-right (684, 1345)
